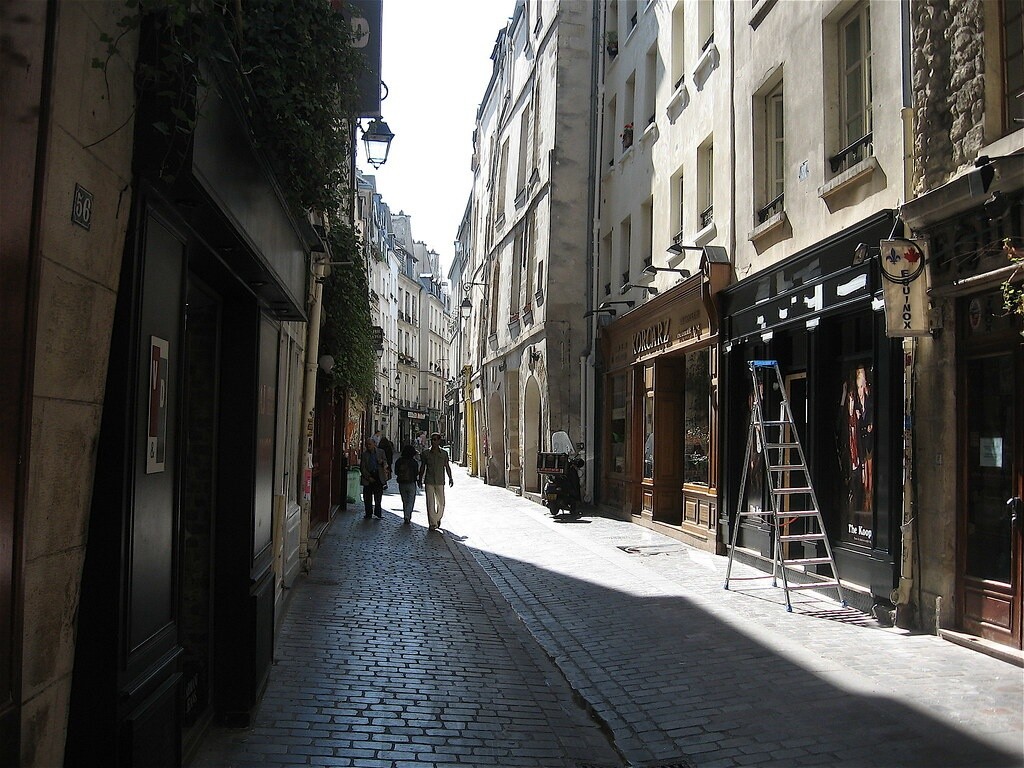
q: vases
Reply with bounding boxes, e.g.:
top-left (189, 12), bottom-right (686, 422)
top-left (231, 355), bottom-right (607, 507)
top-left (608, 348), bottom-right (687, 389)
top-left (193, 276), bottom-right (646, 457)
top-left (523, 302), bottom-right (531, 313)
top-left (622, 137), bottom-right (633, 148)
top-left (694, 444), bottom-right (702, 454)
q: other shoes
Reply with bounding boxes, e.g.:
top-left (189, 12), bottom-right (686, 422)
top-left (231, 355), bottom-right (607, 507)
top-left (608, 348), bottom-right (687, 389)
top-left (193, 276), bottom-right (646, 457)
top-left (374, 513), bottom-right (385, 518)
top-left (429, 524), bottom-right (437, 531)
top-left (364, 515), bottom-right (372, 518)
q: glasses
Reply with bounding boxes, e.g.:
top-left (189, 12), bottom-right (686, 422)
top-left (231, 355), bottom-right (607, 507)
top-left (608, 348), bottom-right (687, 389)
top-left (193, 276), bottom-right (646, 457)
top-left (430, 438), bottom-right (436, 441)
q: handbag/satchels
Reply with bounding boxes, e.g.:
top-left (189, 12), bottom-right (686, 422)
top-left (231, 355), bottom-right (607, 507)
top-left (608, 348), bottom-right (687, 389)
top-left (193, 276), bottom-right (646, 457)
top-left (384, 470), bottom-right (390, 481)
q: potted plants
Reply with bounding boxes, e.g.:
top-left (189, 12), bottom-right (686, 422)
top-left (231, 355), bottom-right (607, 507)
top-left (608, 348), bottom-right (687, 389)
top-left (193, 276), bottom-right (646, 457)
top-left (601, 31), bottom-right (618, 55)
top-left (510, 312), bottom-right (518, 321)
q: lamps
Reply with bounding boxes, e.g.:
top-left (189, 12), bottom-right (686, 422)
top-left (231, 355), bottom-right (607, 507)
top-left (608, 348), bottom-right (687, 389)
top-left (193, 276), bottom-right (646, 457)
top-left (531, 351), bottom-right (541, 362)
top-left (642, 266), bottom-right (690, 278)
top-left (435, 359), bottom-right (449, 377)
top-left (583, 309), bottom-right (616, 318)
top-left (851, 243), bottom-right (880, 267)
top-left (384, 367), bottom-right (401, 384)
top-left (975, 152), bottom-right (1024, 193)
top-left (377, 345), bottom-right (384, 360)
top-left (358, 116), bottom-right (396, 170)
top-left (499, 362), bottom-right (507, 372)
top-left (667, 244), bottom-right (703, 255)
top-left (599, 301), bottom-right (635, 309)
top-left (620, 282), bottom-right (658, 295)
top-left (460, 282), bottom-right (488, 320)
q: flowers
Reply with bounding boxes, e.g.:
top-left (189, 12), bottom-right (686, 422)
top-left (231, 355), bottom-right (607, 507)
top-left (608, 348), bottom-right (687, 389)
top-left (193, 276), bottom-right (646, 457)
top-left (686, 427), bottom-right (709, 444)
top-left (619, 122), bottom-right (634, 138)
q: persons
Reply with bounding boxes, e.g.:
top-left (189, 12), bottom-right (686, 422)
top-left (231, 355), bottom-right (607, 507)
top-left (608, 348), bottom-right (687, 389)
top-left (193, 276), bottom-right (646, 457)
top-left (374, 430), bottom-right (427, 479)
top-left (419, 432), bottom-right (453, 531)
top-left (394, 444), bottom-right (419, 524)
top-left (360, 438), bottom-right (388, 521)
top-left (847, 368), bottom-right (874, 514)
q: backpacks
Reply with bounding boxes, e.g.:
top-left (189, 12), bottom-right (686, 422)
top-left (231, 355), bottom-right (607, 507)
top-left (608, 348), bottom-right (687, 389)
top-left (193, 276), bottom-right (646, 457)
top-left (399, 457), bottom-right (413, 481)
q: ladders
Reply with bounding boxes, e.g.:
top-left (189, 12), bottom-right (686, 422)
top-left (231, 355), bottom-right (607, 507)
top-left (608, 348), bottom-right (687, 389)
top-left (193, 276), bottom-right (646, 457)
top-left (723, 359), bottom-right (846, 612)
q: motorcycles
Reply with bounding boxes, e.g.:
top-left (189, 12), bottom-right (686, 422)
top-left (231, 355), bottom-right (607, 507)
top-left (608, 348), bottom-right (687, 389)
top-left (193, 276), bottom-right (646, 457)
top-left (537, 432), bottom-right (586, 516)
